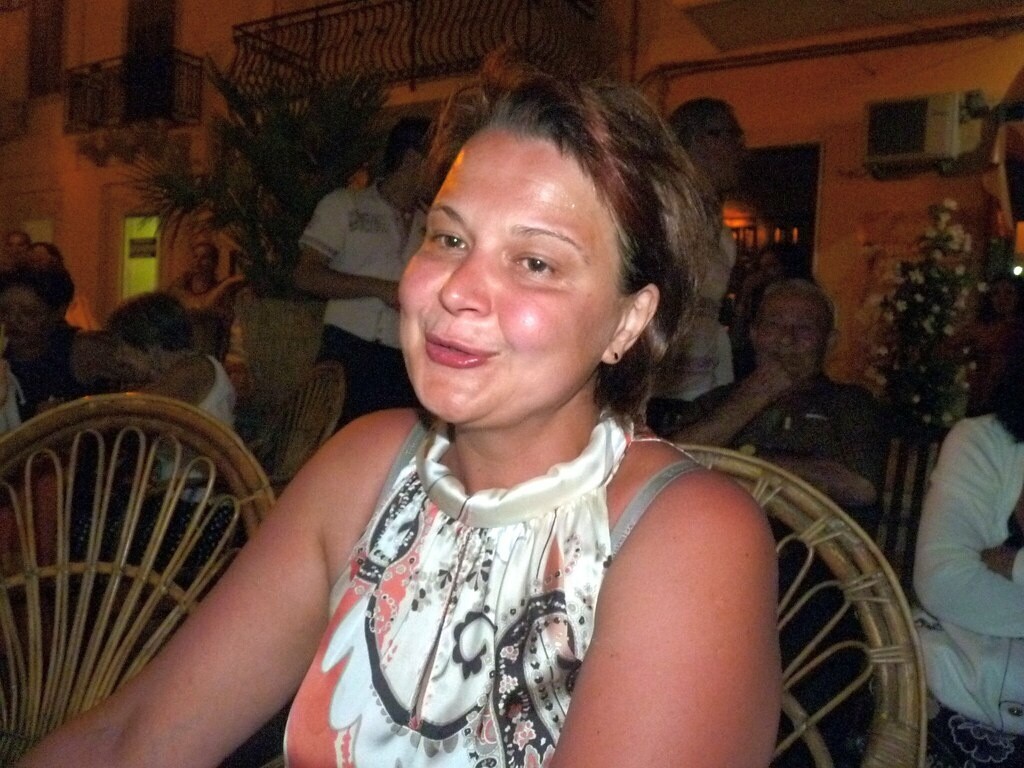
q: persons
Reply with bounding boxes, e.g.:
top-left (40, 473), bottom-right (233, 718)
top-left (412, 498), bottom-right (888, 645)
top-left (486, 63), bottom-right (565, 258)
top-left (0, 49), bottom-right (780, 768)
top-left (670, 279), bottom-right (1024, 768)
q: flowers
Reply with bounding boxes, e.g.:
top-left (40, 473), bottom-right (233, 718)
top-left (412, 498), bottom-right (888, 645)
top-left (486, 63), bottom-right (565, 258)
top-left (860, 199), bottom-right (990, 428)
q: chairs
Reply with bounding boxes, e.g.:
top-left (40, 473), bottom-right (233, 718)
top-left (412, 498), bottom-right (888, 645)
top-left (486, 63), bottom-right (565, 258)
top-left (667, 444), bottom-right (930, 768)
top-left (878, 423), bottom-right (949, 600)
top-left (0, 391), bottom-right (277, 767)
top-left (148, 360), bottom-right (346, 499)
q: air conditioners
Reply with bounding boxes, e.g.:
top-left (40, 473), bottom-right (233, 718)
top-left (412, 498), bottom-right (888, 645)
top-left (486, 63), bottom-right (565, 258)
top-left (860, 91), bottom-right (963, 167)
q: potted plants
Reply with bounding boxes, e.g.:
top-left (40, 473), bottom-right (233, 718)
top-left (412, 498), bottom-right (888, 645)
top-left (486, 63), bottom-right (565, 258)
top-left (110, 35), bottom-right (394, 395)
